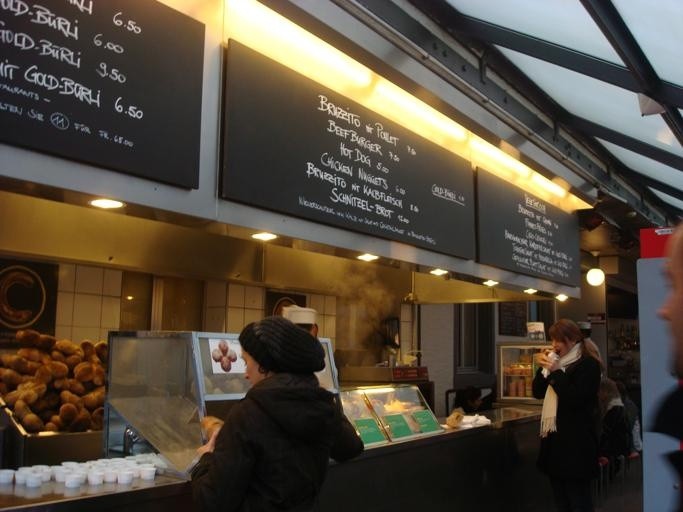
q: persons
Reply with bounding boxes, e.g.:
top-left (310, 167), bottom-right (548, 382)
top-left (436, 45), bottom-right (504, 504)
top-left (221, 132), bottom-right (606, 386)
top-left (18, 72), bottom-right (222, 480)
top-left (452, 387), bottom-right (484, 415)
top-left (530, 318), bottom-right (643, 512)
top-left (644, 219), bottom-right (683, 512)
top-left (188, 315), bottom-right (363, 512)
top-left (282, 304), bottom-right (319, 339)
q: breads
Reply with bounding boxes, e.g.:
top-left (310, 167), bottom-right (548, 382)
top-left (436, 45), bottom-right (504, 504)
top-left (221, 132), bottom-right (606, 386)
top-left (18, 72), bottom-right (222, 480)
top-left (199, 416), bottom-right (223, 442)
top-left (0, 328), bottom-right (108, 433)
top-left (446, 410), bottom-right (463, 428)
top-left (212, 340), bottom-right (238, 371)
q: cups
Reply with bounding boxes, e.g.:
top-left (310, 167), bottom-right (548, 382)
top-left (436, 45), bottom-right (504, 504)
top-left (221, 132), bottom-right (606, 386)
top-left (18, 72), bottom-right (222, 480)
top-left (0, 452), bottom-right (166, 490)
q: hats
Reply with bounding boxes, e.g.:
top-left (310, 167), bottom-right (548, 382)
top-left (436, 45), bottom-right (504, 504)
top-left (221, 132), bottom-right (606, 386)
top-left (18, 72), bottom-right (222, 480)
top-left (239, 305), bottom-right (325, 373)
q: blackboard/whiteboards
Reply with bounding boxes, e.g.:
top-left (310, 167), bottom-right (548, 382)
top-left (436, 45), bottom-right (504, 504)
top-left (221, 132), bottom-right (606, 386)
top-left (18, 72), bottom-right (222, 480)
top-left (474, 167), bottom-right (581, 286)
top-left (1, 0), bottom-right (206, 191)
top-left (218, 39), bottom-right (476, 263)
